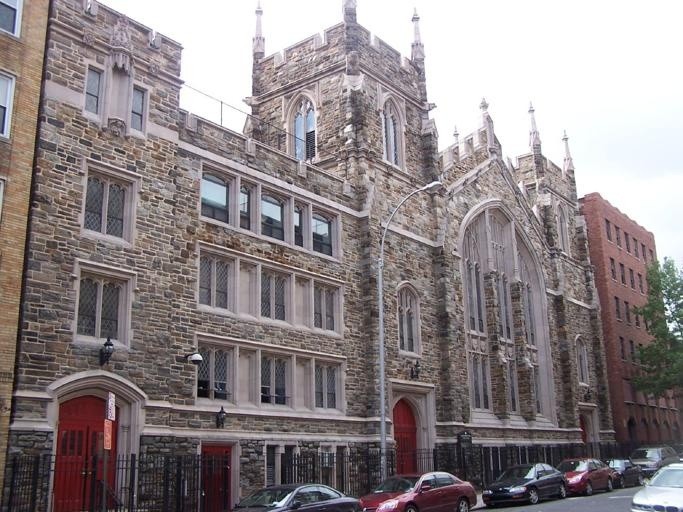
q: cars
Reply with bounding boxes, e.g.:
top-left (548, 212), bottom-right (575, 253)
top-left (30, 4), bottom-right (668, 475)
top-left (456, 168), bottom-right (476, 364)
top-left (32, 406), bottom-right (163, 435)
top-left (553, 456), bottom-right (617, 498)
top-left (626, 462), bottom-right (683, 512)
top-left (225, 482), bottom-right (362, 512)
top-left (479, 462), bottom-right (568, 509)
top-left (604, 455), bottom-right (646, 489)
top-left (359, 469), bottom-right (479, 512)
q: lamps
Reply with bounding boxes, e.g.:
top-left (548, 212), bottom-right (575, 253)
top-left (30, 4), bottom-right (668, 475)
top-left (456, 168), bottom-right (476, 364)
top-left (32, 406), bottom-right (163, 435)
top-left (213, 404), bottom-right (227, 428)
top-left (581, 385), bottom-right (593, 403)
top-left (407, 357), bottom-right (422, 380)
top-left (94, 333), bottom-right (114, 368)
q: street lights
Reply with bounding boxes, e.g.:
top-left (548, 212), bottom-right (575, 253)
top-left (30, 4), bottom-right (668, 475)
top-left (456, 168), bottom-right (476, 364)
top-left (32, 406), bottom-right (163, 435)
top-left (375, 179), bottom-right (444, 481)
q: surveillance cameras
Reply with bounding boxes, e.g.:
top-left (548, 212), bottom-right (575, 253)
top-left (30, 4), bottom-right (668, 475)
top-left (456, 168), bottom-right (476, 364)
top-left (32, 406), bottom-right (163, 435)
top-left (191, 354), bottom-right (203, 364)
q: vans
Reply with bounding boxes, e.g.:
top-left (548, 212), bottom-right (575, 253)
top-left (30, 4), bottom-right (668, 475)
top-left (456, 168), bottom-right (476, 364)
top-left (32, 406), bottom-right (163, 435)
top-left (628, 440), bottom-right (680, 477)
